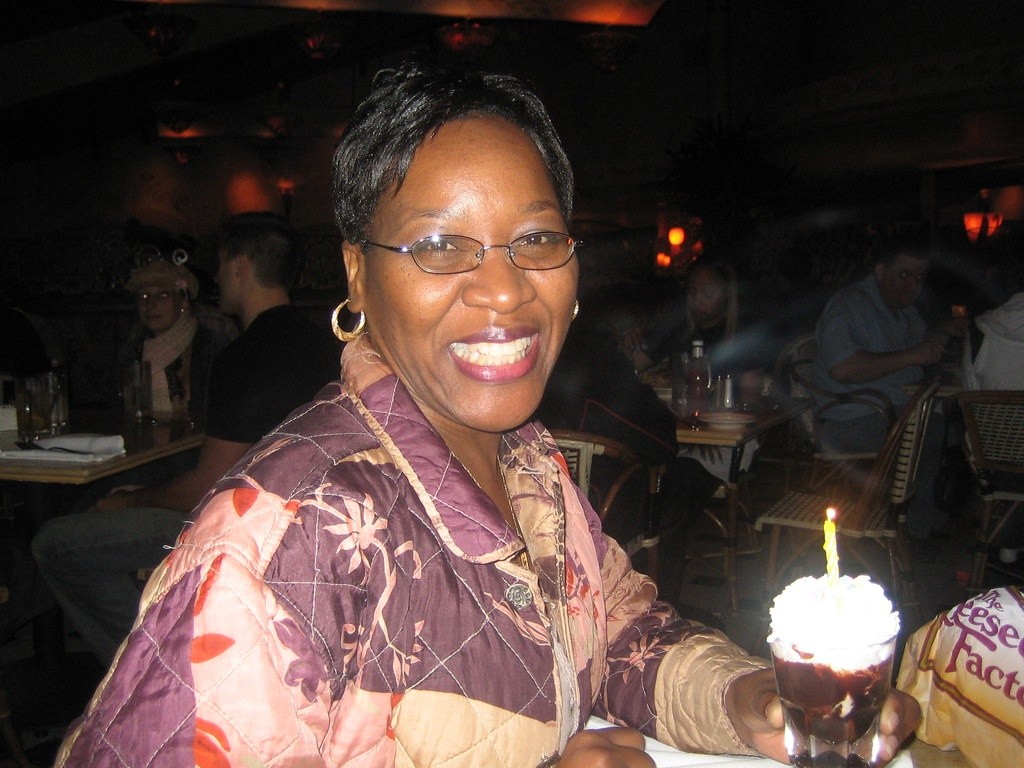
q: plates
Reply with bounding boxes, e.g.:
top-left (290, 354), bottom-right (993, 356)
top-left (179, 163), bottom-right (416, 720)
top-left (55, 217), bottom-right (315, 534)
top-left (653, 388), bottom-right (673, 399)
top-left (698, 412), bottom-right (756, 428)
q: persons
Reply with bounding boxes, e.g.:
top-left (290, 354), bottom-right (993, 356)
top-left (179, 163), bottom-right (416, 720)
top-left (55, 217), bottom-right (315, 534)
top-left (0, 210), bottom-right (341, 740)
top-left (52, 61), bottom-right (924, 768)
top-left (549, 184), bottom-right (1024, 606)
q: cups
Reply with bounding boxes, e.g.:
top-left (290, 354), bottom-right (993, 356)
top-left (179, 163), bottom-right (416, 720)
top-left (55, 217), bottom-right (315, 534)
top-left (50, 374), bottom-right (70, 432)
top-left (768, 634), bottom-right (896, 768)
top-left (16, 375), bottom-right (52, 440)
top-left (124, 361), bottom-right (154, 424)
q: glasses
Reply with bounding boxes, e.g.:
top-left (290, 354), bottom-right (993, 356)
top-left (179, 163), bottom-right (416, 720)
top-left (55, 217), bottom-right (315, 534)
top-left (357, 231), bottom-right (589, 274)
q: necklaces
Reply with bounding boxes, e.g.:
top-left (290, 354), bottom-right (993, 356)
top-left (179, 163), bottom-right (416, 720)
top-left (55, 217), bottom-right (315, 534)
top-left (452, 451), bottom-right (529, 571)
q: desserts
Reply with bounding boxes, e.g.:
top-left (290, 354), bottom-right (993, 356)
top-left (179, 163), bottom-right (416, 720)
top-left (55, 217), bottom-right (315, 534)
top-left (767, 574), bottom-right (900, 744)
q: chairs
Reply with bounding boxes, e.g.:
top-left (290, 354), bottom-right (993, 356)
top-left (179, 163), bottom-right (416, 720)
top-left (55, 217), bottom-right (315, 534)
top-left (957, 389), bottom-right (1024, 599)
top-left (549, 426), bottom-right (668, 593)
top-left (122, 304), bottom-right (242, 411)
top-left (756, 376), bottom-right (943, 629)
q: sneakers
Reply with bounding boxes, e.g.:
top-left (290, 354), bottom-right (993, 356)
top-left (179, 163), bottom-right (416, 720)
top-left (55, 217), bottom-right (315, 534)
top-left (999, 547), bottom-right (1024, 563)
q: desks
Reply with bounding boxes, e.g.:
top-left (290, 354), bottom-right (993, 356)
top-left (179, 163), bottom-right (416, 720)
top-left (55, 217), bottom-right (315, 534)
top-left (675, 396), bottom-right (817, 615)
top-left (0, 407), bottom-right (206, 485)
top-left (901, 370), bottom-right (962, 397)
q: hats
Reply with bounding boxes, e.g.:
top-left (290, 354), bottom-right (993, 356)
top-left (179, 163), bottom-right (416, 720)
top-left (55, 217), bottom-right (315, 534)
top-left (134, 260), bottom-right (199, 301)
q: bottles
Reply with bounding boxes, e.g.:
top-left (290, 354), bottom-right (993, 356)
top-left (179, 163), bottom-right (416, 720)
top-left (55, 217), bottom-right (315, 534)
top-left (713, 375), bottom-right (723, 410)
top-left (724, 375), bottom-right (734, 409)
top-left (672, 375), bottom-right (689, 418)
top-left (688, 340), bottom-right (709, 429)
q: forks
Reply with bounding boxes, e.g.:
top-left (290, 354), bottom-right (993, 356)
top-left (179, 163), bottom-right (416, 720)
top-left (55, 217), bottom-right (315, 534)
top-left (16, 441), bottom-right (94, 455)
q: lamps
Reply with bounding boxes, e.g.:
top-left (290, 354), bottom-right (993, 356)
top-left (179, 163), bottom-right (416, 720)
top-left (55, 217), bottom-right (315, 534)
top-left (576, 26), bottom-right (643, 72)
top-left (437, 22), bottom-right (503, 64)
top-left (121, 14), bottom-right (198, 54)
top-left (289, 18), bottom-right (359, 60)
